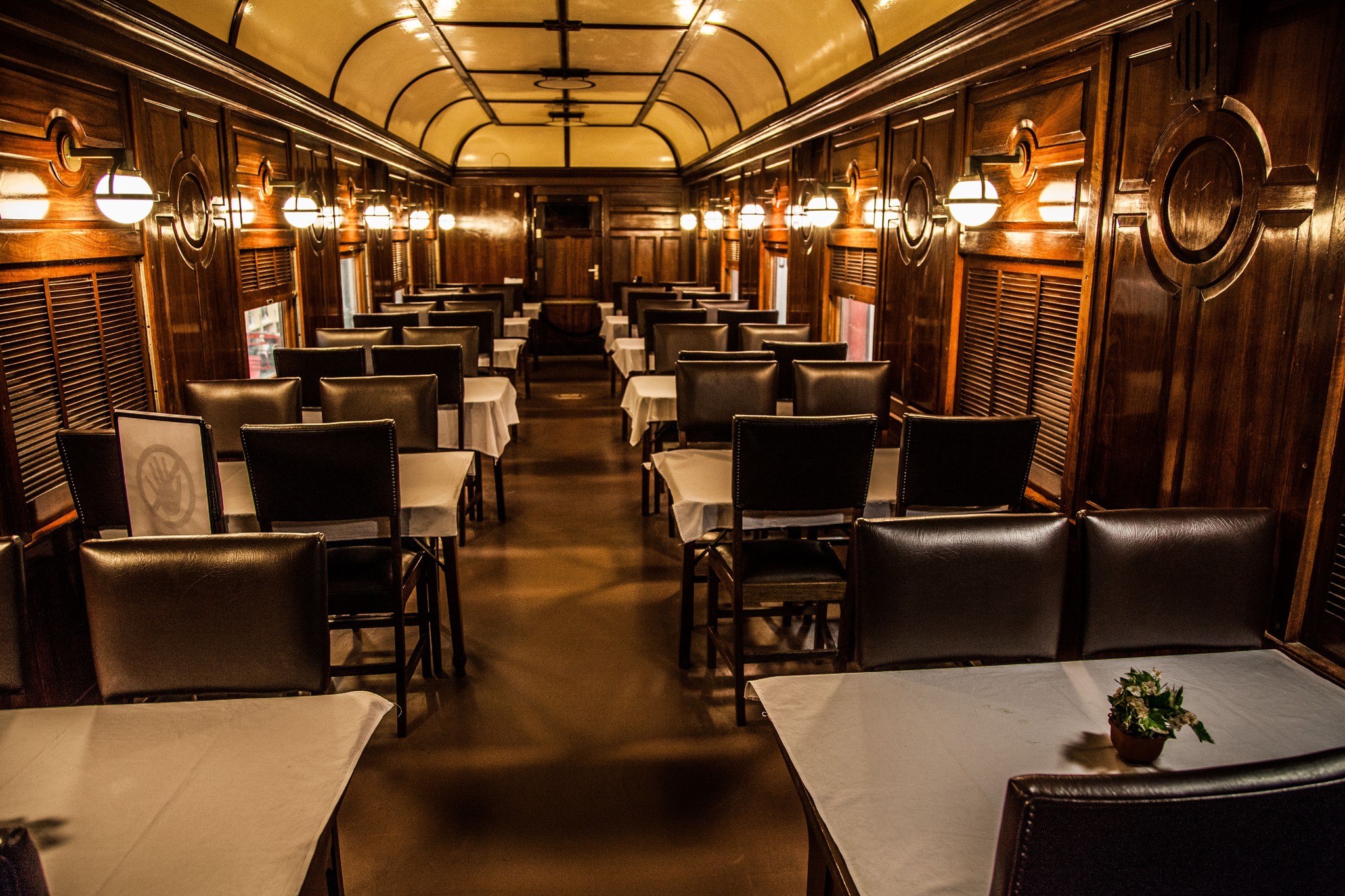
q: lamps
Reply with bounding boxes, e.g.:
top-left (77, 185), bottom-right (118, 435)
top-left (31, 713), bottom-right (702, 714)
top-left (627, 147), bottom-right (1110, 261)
top-left (679, 142), bottom-right (1029, 233)
top-left (57, 134), bottom-right (454, 232)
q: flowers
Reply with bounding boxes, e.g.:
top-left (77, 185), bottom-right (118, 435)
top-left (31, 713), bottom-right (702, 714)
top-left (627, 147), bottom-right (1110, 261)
top-left (1106, 664), bottom-right (1216, 746)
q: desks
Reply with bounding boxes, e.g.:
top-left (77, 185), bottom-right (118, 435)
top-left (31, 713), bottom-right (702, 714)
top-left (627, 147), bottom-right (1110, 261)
top-left (296, 376), bottom-right (519, 527)
top-left (502, 317), bottom-right (532, 400)
top-left (515, 299), bottom-right (543, 372)
top-left (101, 449), bottom-right (476, 681)
top-left (478, 338), bottom-right (527, 445)
top-left (743, 650), bottom-right (1345, 895)
top-left (0, 691), bottom-right (395, 896)
top-left (598, 302), bottom-right (622, 369)
top-left (613, 337), bottom-right (655, 441)
top-left (619, 373), bottom-right (794, 514)
top-left (599, 315), bottom-right (638, 396)
top-left (651, 441), bottom-right (1004, 670)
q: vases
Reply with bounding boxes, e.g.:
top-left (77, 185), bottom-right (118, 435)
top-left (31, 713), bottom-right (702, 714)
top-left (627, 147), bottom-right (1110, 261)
top-left (1108, 718), bottom-right (1166, 763)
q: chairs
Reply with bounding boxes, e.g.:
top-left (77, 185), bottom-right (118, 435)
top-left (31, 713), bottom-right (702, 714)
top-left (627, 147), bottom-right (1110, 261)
top-left (609, 276), bottom-right (1345, 896)
top-left (56, 280), bottom-right (524, 896)
